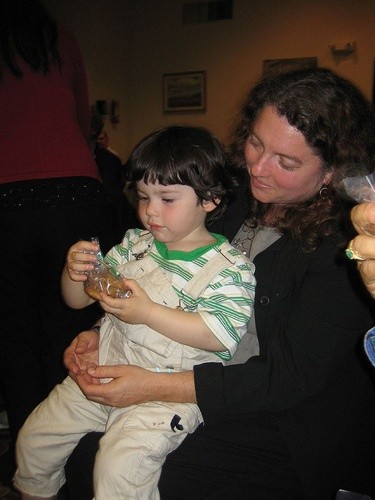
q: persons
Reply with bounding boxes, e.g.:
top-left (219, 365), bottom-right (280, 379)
top-left (0, 1), bottom-right (103, 433)
top-left (9, 124), bottom-right (258, 499)
top-left (62, 63), bottom-right (375, 500)
top-left (343, 180), bottom-right (375, 380)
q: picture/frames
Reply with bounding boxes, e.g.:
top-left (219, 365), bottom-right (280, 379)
top-left (262, 57), bottom-right (318, 79)
top-left (163, 71), bottom-right (206, 112)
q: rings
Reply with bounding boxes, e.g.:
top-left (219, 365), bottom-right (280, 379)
top-left (345, 239), bottom-right (365, 261)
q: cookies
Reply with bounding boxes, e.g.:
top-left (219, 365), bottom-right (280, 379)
top-left (85, 272), bottom-right (130, 301)
